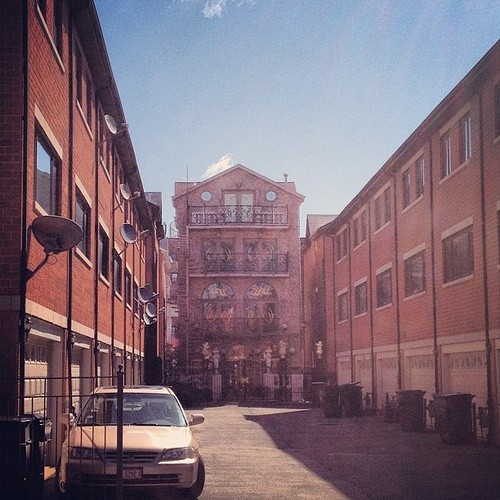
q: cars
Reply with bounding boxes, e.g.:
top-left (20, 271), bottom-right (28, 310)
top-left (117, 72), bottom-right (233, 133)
top-left (56, 385), bottom-right (206, 499)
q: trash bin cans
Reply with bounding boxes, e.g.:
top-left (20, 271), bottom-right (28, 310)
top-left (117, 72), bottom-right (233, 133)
top-left (320, 384), bottom-right (340, 419)
top-left (396, 390), bottom-right (426, 429)
top-left (434, 393), bottom-right (479, 442)
top-left (336, 385), bottom-right (368, 414)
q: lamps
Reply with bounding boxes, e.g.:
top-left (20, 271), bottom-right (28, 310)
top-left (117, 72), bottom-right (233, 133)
top-left (97, 341), bottom-right (100, 353)
top-left (125, 350), bottom-right (143, 363)
top-left (25, 317), bottom-right (32, 342)
top-left (113, 346), bottom-right (116, 356)
top-left (70, 333), bottom-right (76, 351)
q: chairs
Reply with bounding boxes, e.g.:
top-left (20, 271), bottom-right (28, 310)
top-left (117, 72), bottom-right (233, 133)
top-left (96, 400), bottom-right (117, 423)
top-left (138, 401), bottom-right (165, 424)
